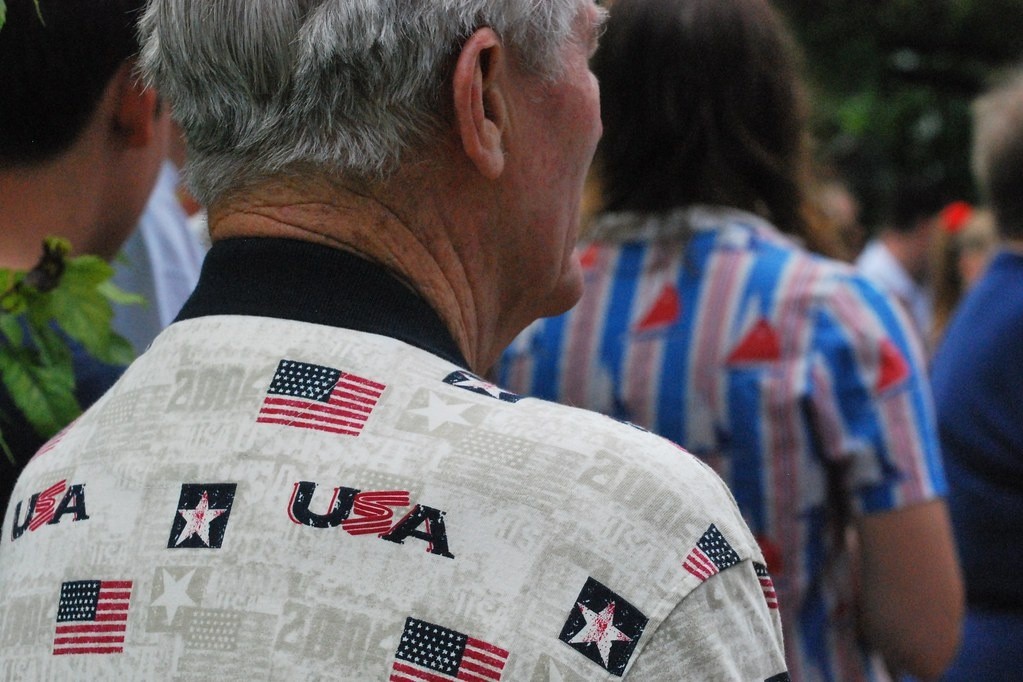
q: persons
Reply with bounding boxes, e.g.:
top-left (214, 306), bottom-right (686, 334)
top-left (0, 0), bottom-right (1023, 682)
